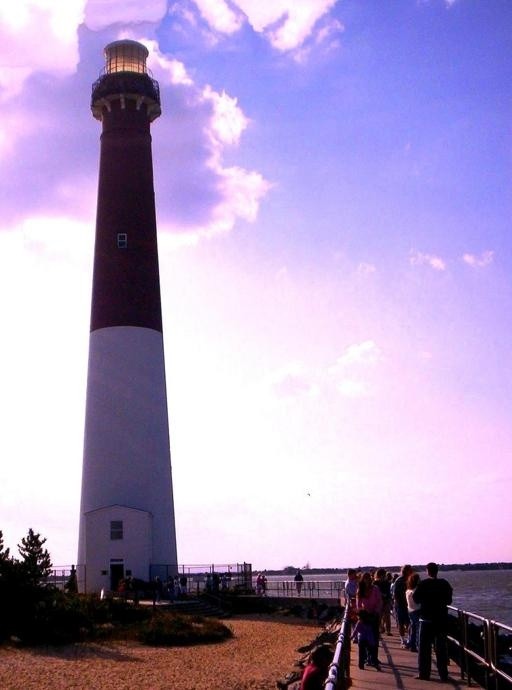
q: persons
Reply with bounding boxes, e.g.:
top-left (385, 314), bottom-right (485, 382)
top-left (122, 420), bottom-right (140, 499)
top-left (293, 571), bottom-right (303, 597)
top-left (344, 564), bottom-right (422, 670)
top-left (220, 572), bottom-right (229, 591)
top-left (413, 561), bottom-right (454, 682)
top-left (259, 575), bottom-right (268, 594)
top-left (118, 572), bottom-right (189, 607)
top-left (255, 573), bottom-right (263, 595)
top-left (294, 644), bottom-right (335, 690)
